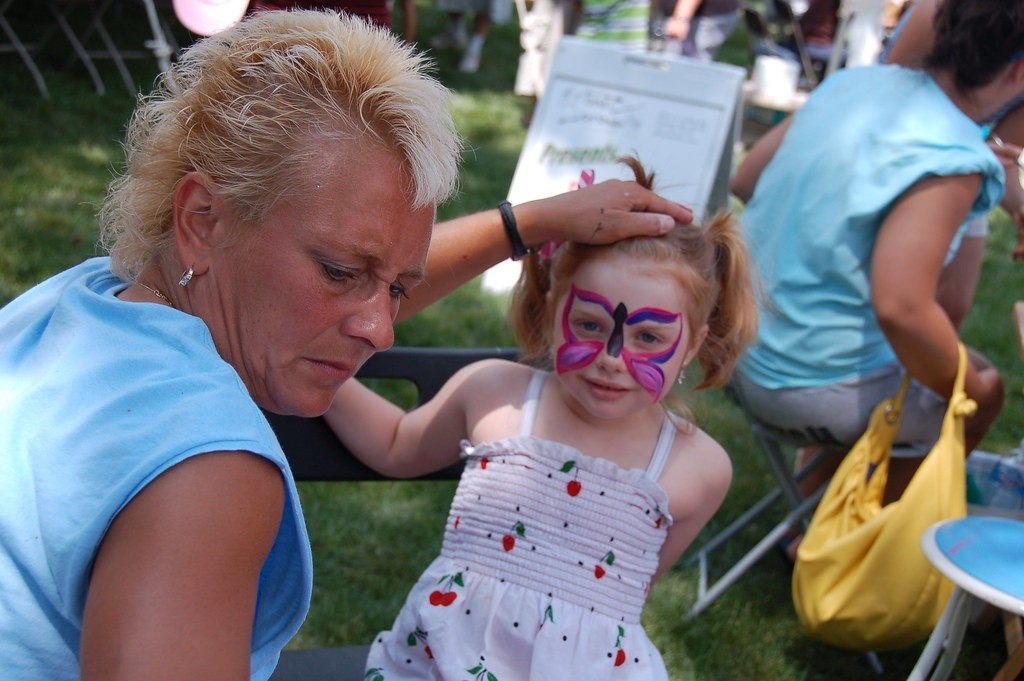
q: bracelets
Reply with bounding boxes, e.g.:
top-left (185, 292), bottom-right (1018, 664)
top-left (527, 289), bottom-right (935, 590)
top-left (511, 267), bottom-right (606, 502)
top-left (498, 201), bottom-right (529, 260)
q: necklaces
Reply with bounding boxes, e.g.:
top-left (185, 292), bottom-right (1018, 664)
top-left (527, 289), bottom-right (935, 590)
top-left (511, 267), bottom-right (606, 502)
top-left (130, 279), bottom-right (173, 305)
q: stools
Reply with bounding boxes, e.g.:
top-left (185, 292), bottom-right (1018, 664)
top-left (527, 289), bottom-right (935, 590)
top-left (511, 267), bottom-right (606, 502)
top-left (905, 516), bottom-right (1024, 681)
top-left (671, 407), bottom-right (925, 677)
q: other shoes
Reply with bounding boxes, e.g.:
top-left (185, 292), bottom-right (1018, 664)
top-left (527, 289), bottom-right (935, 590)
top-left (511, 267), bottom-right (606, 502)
top-left (431, 32), bottom-right (468, 49)
top-left (460, 47), bottom-right (480, 73)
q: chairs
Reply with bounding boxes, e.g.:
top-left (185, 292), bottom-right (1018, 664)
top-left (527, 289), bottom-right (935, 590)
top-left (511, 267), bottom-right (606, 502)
top-left (259, 347), bottom-right (519, 681)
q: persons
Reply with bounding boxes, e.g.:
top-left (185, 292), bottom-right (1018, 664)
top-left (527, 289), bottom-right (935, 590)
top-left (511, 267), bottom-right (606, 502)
top-left (0, 11), bottom-right (693, 681)
top-left (244, 0), bottom-right (415, 43)
top-left (728, 1), bottom-right (1024, 568)
top-left (326, 156), bottom-right (755, 681)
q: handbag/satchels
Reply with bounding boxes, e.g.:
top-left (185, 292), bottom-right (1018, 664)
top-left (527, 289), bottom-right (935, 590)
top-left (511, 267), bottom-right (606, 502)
top-left (791, 342), bottom-right (977, 652)
